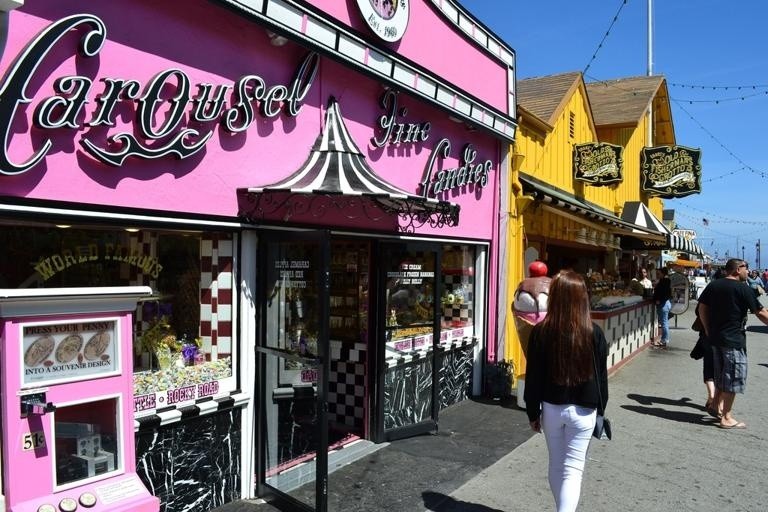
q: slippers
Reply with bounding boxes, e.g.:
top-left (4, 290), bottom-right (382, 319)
top-left (705, 400), bottom-right (747, 430)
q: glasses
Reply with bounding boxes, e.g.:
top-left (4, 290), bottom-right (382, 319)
top-left (738, 262), bottom-right (749, 269)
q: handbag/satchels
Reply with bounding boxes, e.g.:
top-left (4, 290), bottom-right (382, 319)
top-left (591, 413), bottom-right (612, 440)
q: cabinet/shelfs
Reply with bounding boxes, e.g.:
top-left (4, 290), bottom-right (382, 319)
top-left (267, 240), bottom-right (360, 337)
top-left (360, 242), bottom-right (398, 286)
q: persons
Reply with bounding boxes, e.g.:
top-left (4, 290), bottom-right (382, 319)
top-left (626, 266), bottom-right (652, 296)
top-left (654, 267), bottom-right (673, 347)
top-left (523, 268), bottom-right (609, 512)
top-left (685, 258), bottom-right (767, 429)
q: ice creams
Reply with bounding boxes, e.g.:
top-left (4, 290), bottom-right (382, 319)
top-left (511, 260), bottom-right (555, 360)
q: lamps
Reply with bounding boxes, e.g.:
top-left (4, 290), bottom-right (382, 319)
top-left (565, 226), bottom-right (623, 251)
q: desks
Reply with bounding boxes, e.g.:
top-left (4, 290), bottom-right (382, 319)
top-left (590, 296), bottom-right (659, 379)
